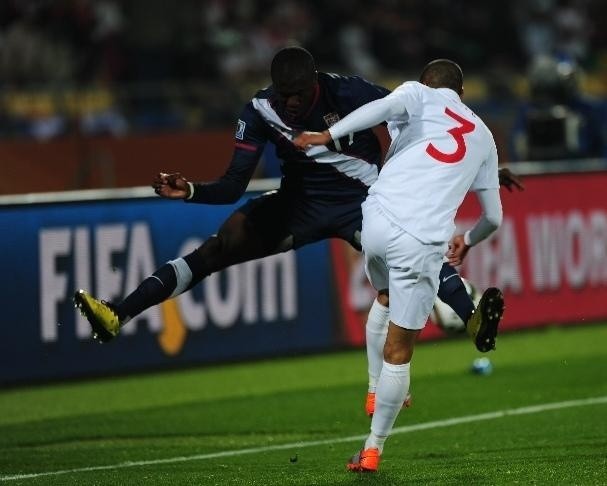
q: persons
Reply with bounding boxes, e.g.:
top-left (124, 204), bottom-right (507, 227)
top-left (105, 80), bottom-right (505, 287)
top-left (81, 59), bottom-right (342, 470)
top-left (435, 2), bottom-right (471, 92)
top-left (0, 0), bottom-right (607, 162)
top-left (73, 46), bottom-right (525, 353)
top-left (292, 58), bottom-right (503, 471)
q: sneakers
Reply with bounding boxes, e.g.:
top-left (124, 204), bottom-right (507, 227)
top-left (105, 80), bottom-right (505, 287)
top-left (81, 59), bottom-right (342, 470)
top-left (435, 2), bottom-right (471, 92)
top-left (467, 287), bottom-right (506, 353)
top-left (365, 392), bottom-right (411, 416)
top-left (72, 288), bottom-right (120, 344)
top-left (347, 446), bottom-right (380, 472)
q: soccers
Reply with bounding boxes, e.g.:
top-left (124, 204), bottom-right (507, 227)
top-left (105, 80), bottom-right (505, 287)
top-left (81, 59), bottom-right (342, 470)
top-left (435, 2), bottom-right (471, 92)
top-left (428, 278), bottom-right (483, 334)
